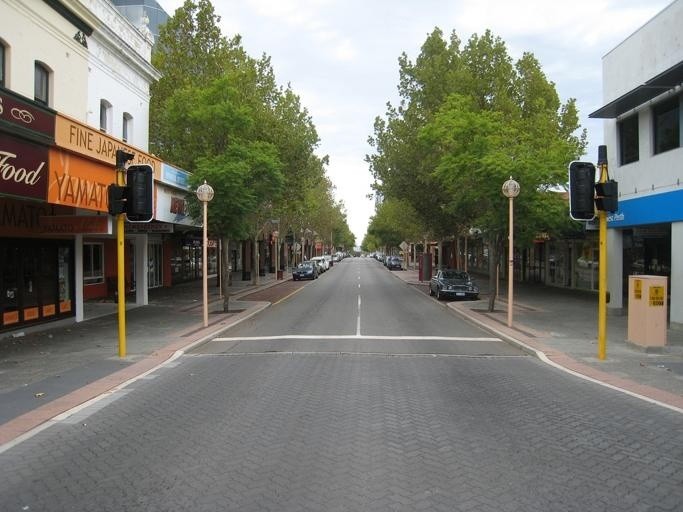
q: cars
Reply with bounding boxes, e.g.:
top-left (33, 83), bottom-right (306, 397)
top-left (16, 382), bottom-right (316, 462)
top-left (293, 248), bottom-right (351, 280)
top-left (354, 251), bottom-right (405, 272)
top-left (167, 250), bottom-right (233, 278)
top-left (429, 268), bottom-right (481, 301)
top-left (546, 251), bottom-right (599, 270)
top-left (632, 256), bottom-right (671, 275)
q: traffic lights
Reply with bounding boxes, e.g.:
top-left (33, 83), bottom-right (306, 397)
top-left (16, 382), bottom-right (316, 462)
top-left (593, 180), bottom-right (617, 213)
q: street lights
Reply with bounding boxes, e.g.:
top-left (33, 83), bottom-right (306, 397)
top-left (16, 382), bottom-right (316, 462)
top-left (197, 179), bottom-right (217, 328)
top-left (501, 173), bottom-right (523, 329)
